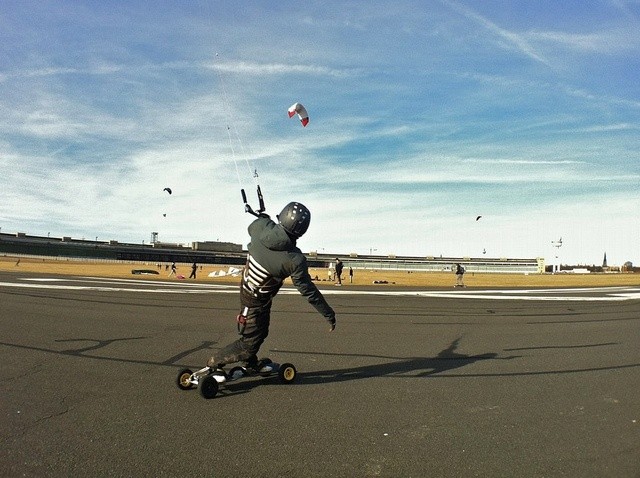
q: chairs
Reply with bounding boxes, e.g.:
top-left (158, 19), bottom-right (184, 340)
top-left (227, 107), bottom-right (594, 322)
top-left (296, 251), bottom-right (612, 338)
top-left (247, 361), bottom-right (273, 373)
top-left (210, 367), bottom-right (226, 383)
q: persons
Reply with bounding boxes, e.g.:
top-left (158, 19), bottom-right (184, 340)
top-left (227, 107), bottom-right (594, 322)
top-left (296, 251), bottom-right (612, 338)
top-left (205, 201), bottom-right (336, 385)
top-left (454, 263), bottom-right (464, 286)
top-left (350, 267), bottom-right (353, 283)
top-left (189, 261), bottom-right (198, 279)
top-left (335, 258), bottom-right (344, 286)
top-left (168, 261), bottom-right (179, 278)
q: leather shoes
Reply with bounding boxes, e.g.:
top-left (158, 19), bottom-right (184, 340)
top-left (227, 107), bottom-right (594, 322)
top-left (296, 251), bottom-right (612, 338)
top-left (259, 213), bottom-right (270, 218)
top-left (326, 315), bottom-right (336, 330)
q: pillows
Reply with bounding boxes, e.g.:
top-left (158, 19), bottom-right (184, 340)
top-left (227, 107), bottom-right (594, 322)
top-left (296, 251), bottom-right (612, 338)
top-left (277, 202), bottom-right (310, 238)
